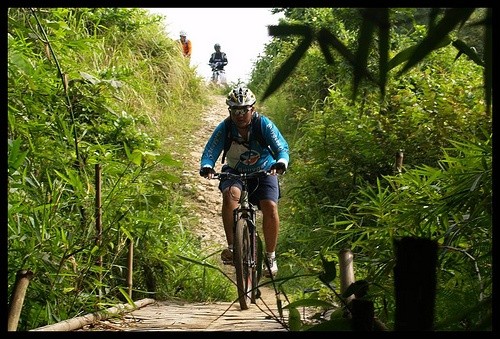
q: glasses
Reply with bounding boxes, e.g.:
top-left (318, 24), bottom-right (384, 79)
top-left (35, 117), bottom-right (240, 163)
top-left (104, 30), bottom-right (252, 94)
top-left (228, 107), bottom-right (252, 116)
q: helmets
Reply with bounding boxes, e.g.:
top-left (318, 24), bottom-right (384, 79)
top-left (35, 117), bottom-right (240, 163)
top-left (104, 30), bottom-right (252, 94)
top-left (214, 44), bottom-right (221, 48)
top-left (179, 30), bottom-right (188, 36)
top-left (225, 83), bottom-right (257, 108)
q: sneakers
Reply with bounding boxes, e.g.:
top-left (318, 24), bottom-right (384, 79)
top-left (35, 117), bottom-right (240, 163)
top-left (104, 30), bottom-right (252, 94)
top-left (221, 248), bottom-right (235, 266)
top-left (263, 257), bottom-right (278, 276)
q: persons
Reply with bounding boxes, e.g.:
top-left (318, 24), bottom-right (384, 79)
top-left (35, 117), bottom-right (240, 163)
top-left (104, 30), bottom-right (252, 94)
top-left (208, 44), bottom-right (227, 83)
top-left (177, 31), bottom-right (192, 59)
top-left (200, 86), bottom-right (289, 277)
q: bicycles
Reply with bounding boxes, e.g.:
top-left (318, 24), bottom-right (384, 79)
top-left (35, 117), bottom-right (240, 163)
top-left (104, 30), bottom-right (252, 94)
top-left (201, 168), bottom-right (289, 310)
